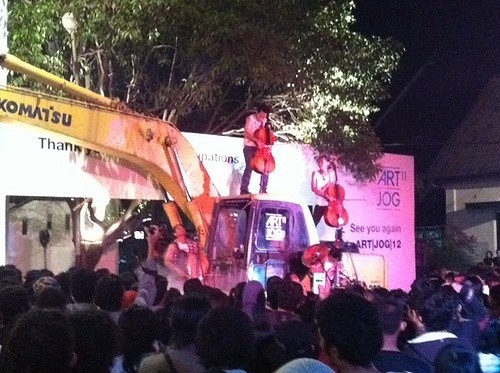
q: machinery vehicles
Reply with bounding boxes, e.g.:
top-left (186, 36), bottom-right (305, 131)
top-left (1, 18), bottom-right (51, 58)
top-left (0, 52), bottom-right (324, 312)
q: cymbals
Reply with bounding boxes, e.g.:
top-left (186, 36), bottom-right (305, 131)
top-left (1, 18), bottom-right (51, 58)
top-left (300, 244), bottom-right (330, 267)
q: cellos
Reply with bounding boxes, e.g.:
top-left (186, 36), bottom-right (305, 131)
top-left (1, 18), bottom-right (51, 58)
top-left (183, 226), bottom-right (209, 285)
top-left (250, 104), bottom-right (277, 173)
top-left (323, 158), bottom-right (349, 228)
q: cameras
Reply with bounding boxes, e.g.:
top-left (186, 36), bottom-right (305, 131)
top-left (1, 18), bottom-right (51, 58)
top-left (134, 218), bottom-right (153, 239)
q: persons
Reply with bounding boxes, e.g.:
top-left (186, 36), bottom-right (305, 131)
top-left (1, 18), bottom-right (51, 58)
top-left (311, 155), bottom-right (344, 243)
top-left (1, 224), bottom-right (500, 373)
top-left (240, 104), bottom-right (277, 195)
